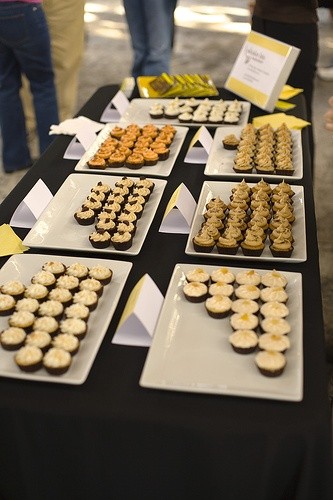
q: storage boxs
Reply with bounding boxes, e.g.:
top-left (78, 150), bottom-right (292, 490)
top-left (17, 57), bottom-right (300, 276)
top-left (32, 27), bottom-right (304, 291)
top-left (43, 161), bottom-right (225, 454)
top-left (223, 30), bottom-right (302, 113)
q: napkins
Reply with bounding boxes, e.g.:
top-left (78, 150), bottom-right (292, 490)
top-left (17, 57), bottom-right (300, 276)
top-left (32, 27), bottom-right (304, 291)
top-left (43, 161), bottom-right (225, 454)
top-left (48, 116), bottom-right (104, 135)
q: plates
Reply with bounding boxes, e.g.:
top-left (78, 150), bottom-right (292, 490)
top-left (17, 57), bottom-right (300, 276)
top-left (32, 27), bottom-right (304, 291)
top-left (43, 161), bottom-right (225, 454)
top-left (138, 263), bottom-right (304, 402)
top-left (137, 74), bottom-right (219, 97)
top-left (0, 254), bottom-right (133, 386)
top-left (118, 97), bottom-right (251, 127)
top-left (22, 173), bottom-right (168, 256)
top-left (203, 126), bottom-right (303, 180)
top-left (184, 180), bottom-right (307, 263)
top-left (73, 122), bottom-right (189, 177)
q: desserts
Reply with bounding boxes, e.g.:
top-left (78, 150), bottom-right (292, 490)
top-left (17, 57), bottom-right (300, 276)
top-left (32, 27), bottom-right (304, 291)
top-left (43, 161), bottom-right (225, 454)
top-left (74, 176), bottom-right (155, 252)
top-left (150, 95), bottom-right (243, 125)
top-left (87, 123), bottom-right (177, 169)
top-left (183, 268), bottom-right (292, 377)
top-left (222, 122), bottom-right (295, 176)
top-left (0, 261), bottom-right (113, 376)
top-left (192, 178), bottom-right (295, 258)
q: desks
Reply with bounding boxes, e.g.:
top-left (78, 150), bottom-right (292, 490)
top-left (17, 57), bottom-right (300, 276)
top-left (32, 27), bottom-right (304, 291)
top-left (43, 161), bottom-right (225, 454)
top-left (0, 84), bottom-right (333, 500)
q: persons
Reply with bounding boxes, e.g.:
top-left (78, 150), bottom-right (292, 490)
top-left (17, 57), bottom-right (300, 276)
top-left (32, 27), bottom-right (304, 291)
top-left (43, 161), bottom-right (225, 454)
top-left (0, 0), bottom-right (59, 174)
top-left (123, 0), bottom-right (178, 77)
top-left (252, 0), bottom-right (319, 182)
